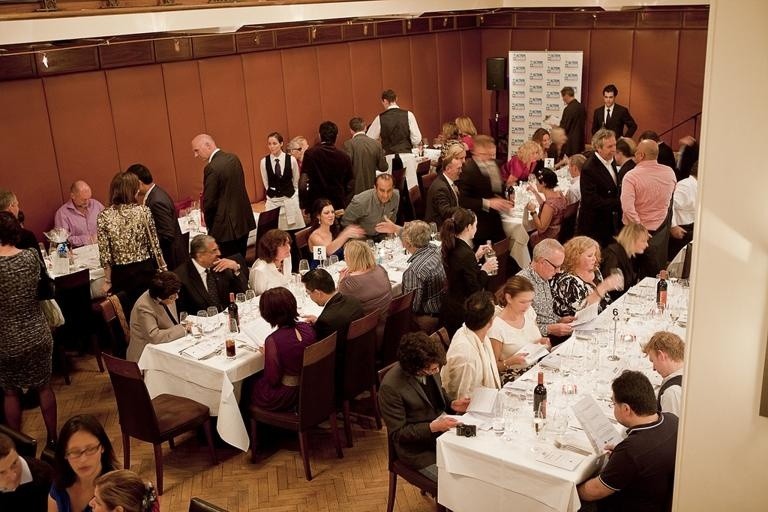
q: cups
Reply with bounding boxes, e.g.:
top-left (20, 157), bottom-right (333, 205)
top-left (236, 292), bottom-right (246, 306)
top-left (225, 339), bottom-right (237, 360)
top-left (246, 290), bottom-right (256, 304)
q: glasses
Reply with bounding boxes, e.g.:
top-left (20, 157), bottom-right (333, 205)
top-left (66, 442), bottom-right (101, 459)
top-left (546, 259), bottom-right (564, 272)
top-left (609, 402), bottom-right (619, 408)
top-left (292, 148), bottom-right (302, 151)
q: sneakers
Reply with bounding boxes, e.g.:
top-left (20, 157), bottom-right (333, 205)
top-left (44, 440), bottom-right (59, 455)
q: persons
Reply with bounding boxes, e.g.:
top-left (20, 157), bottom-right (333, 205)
top-left (551, 87), bottom-right (586, 156)
top-left (56, 90), bottom-right (421, 297)
top-left (380, 130), bottom-right (698, 511)
top-left (126, 173), bottom-right (446, 413)
top-left (591, 85), bottom-right (637, 138)
top-left (0, 415), bottom-right (160, 511)
top-left (1, 191), bottom-right (58, 441)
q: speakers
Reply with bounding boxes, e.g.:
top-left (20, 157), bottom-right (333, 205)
top-left (486, 56), bottom-right (507, 90)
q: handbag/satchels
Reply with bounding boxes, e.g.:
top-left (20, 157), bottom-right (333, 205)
top-left (154, 253), bottom-right (169, 274)
top-left (37, 277), bottom-right (66, 329)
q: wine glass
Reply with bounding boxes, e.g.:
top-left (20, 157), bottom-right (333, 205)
top-left (299, 259), bottom-right (310, 276)
top-left (316, 254), bottom-right (339, 269)
top-left (226, 319), bottom-right (239, 340)
top-left (180, 306), bottom-right (218, 342)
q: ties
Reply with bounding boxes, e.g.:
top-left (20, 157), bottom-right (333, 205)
top-left (606, 109), bottom-right (611, 126)
top-left (275, 159), bottom-right (281, 178)
top-left (205, 268), bottom-right (221, 310)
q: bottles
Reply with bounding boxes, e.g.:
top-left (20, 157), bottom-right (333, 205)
top-left (418, 140), bottom-right (424, 157)
top-left (228, 292), bottom-right (240, 332)
top-left (656, 270), bottom-right (668, 311)
top-left (484, 239), bottom-right (498, 277)
top-left (532, 372), bottom-right (546, 418)
top-left (507, 186), bottom-right (516, 208)
